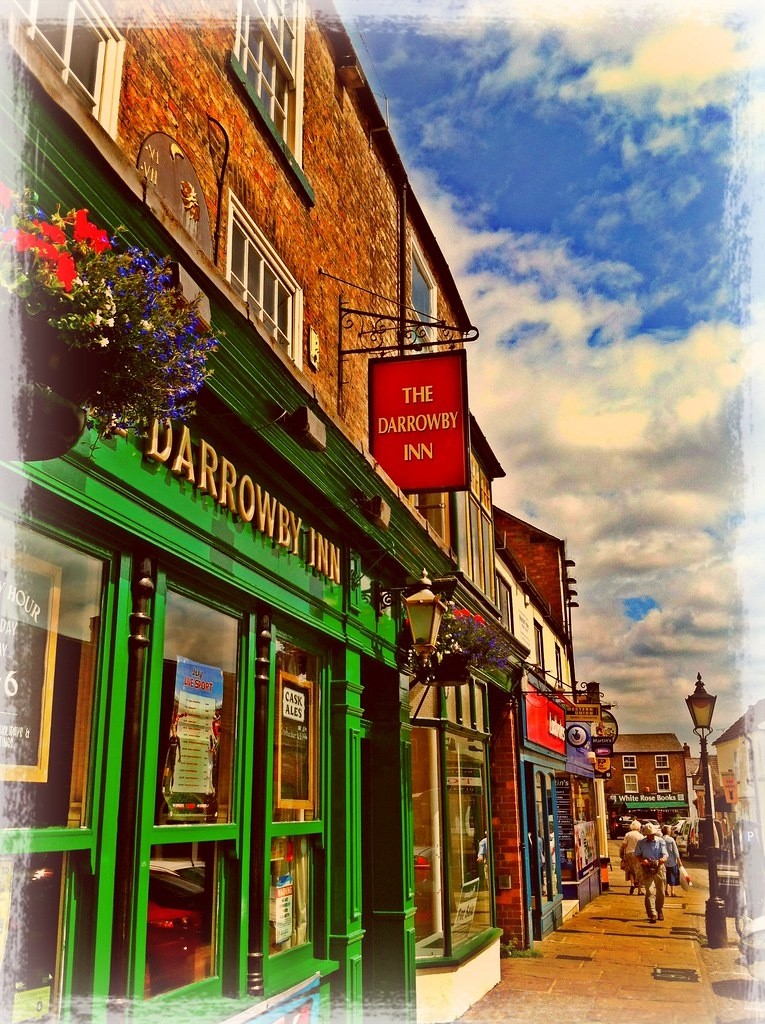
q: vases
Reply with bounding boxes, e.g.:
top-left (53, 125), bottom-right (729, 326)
top-left (2, 304), bottom-right (101, 462)
top-left (416, 653), bottom-right (472, 687)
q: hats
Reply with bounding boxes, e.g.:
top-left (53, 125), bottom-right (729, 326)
top-left (643, 823), bottom-right (657, 835)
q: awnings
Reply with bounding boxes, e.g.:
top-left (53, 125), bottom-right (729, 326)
top-left (625, 801), bottom-right (688, 809)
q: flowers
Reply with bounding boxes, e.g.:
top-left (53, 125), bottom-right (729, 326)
top-left (2, 183), bottom-right (218, 461)
top-left (400, 599), bottom-right (513, 672)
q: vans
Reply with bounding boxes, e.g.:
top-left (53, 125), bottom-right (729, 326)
top-left (674, 818), bottom-right (724, 861)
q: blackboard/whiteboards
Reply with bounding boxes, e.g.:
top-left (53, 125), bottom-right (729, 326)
top-left (277, 669), bottom-right (316, 811)
top-left (0, 542), bottom-right (63, 784)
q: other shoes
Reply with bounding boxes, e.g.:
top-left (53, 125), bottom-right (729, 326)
top-left (664, 891), bottom-right (669, 895)
top-left (658, 910), bottom-right (663, 920)
top-left (630, 886), bottom-right (635, 895)
top-left (650, 915), bottom-right (656, 923)
top-left (671, 893), bottom-right (676, 897)
top-left (638, 892), bottom-right (644, 895)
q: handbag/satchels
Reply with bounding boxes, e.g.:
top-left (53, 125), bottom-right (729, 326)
top-left (620, 859), bottom-right (625, 870)
top-left (678, 866), bottom-right (693, 892)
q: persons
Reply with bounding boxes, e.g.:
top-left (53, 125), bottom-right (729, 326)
top-left (478, 830), bottom-right (488, 881)
top-left (660, 825), bottom-right (682, 897)
top-left (537, 820), bottom-right (556, 896)
top-left (635, 822), bottom-right (667, 922)
top-left (620, 820), bottom-right (645, 895)
top-left (162, 725), bottom-right (181, 795)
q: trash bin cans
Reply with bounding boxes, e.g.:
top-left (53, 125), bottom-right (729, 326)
top-left (717, 864), bottom-right (740, 918)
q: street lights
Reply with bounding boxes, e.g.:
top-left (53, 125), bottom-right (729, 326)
top-left (684, 671), bottom-right (729, 949)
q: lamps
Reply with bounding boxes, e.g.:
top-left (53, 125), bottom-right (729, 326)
top-left (376, 566), bottom-right (449, 669)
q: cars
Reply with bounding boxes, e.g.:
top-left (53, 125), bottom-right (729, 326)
top-left (610, 816), bottom-right (662, 839)
top-left (670, 820), bottom-right (685, 838)
